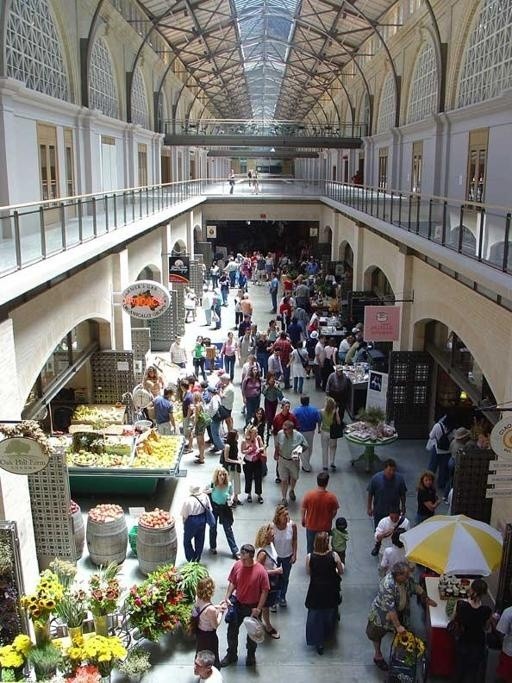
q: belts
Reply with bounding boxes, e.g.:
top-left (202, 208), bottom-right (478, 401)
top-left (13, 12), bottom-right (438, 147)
top-left (280, 455), bottom-right (293, 461)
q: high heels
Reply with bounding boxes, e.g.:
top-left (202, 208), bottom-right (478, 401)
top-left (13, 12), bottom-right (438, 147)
top-left (317, 644), bottom-right (323, 655)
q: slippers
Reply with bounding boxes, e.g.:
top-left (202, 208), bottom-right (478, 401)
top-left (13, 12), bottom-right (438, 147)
top-left (373, 657), bottom-right (389, 673)
top-left (264, 626), bottom-right (280, 640)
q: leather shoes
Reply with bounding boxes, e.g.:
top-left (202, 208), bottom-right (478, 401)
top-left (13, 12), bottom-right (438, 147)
top-left (246, 650), bottom-right (257, 666)
top-left (220, 654), bottom-right (239, 667)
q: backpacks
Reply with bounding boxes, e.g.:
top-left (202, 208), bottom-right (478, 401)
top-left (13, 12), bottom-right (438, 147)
top-left (243, 612), bottom-right (266, 645)
top-left (435, 424), bottom-right (454, 451)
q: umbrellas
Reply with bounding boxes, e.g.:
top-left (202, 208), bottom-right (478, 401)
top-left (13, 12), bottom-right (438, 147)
top-left (398, 514), bottom-right (507, 577)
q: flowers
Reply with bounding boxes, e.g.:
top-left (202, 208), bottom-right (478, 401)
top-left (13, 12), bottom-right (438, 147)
top-left (394, 630), bottom-right (425, 668)
top-left (85, 634), bottom-right (127, 676)
top-left (1, 633), bottom-right (32, 667)
top-left (40, 569), bottom-right (65, 602)
top-left (131, 564), bottom-right (183, 626)
top-left (20, 589), bottom-right (54, 640)
top-left (138, 604), bottom-right (191, 648)
top-left (54, 635), bottom-right (86, 676)
top-left (78, 576), bottom-right (119, 617)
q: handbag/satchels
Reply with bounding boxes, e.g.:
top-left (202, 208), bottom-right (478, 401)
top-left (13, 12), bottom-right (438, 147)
top-left (330, 411), bottom-right (344, 440)
top-left (210, 401), bottom-right (232, 422)
top-left (297, 348), bottom-right (309, 368)
top-left (195, 495), bottom-right (217, 528)
top-left (327, 550), bottom-right (343, 596)
top-left (445, 600), bottom-right (467, 641)
top-left (257, 549), bottom-right (283, 609)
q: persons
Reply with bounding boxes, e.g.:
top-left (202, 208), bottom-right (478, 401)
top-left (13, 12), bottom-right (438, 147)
top-left (219, 271), bottom-right (230, 306)
top-left (177, 375), bottom-right (211, 465)
top-left (208, 261), bottom-right (219, 288)
top-left (495, 604), bottom-right (512, 683)
top-left (193, 650), bottom-right (222, 683)
top-left (268, 505), bottom-right (298, 613)
top-left (271, 400), bottom-right (297, 482)
top-left (220, 332), bottom-right (238, 383)
top-left (451, 580), bottom-right (492, 683)
top-left (351, 170), bottom-right (362, 188)
top-left (301, 473), bottom-right (340, 574)
top-left (169, 334), bottom-right (188, 369)
top-left (213, 289), bottom-right (221, 330)
top-left (214, 369), bottom-right (226, 390)
top-left (220, 544), bottom-right (268, 666)
top-left (445, 425), bottom-right (494, 514)
top-left (379, 528), bottom-right (415, 577)
top-left (240, 353), bottom-right (262, 413)
top-left (185, 289), bottom-right (197, 323)
top-left (254, 525), bottom-right (284, 640)
top-left (195, 579), bottom-right (226, 673)
top-left (426, 412), bottom-right (449, 489)
top-left (241, 427), bottom-right (266, 504)
top-left (338, 328), bottom-right (369, 365)
top-left (415, 473), bottom-right (445, 527)
top-left (244, 407), bottom-right (269, 480)
top-left (265, 264), bottom-right (342, 295)
top-left (203, 287), bottom-right (213, 325)
top-left (234, 289), bottom-right (252, 329)
top-left (202, 338), bottom-right (215, 374)
top-left (365, 562), bottom-right (430, 671)
top-left (292, 396), bottom-right (321, 471)
top-left (325, 365), bottom-right (352, 428)
top-left (286, 340), bottom-right (309, 395)
top-left (206, 387), bottom-right (224, 454)
top-left (191, 336), bottom-right (207, 383)
top-left (203, 469), bottom-right (242, 560)
top-left (132, 366), bottom-right (177, 435)
top-left (266, 250), bottom-right (319, 264)
top-left (313, 335), bottom-right (324, 392)
top-left (267, 295), bottom-right (319, 340)
top-left (262, 373), bottom-right (284, 435)
top-left (324, 338), bottom-right (336, 391)
top-left (330, 517), bottom-right (350, 565)
top-left (367, 458), bottom-right (407, 556)
top-left (267, 332), bottom-right (294, 388)
top-left (318, 396), bottom-right (340, 470)
top-left (240, 367), bottom-right (262, 416)
top-left (182, 484), bottom-right (215, 564)
top-left (223, 253), bottom-right (265, 289)
top-left (221, 431), bottom-right (243, 508)
top-left (304, 531), bottom-right (344, 656)
top-left (305, 332), bottom-right (319, 377)
top-left (274, 420), bottom-right (308, 506)
top-left (227, 168), bottom-right (236, 194)
top-left (238, 316), bottom-right (266, 367)
top-left (265, 346), bottom-right (282, 389)
top-left (373, 506), bottom-right (411, 575)
top-left (220, 373), bottom-right (236, 432)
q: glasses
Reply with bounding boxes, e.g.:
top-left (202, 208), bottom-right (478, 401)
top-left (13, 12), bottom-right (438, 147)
top-left (242, 547), bottom-right (252, 554)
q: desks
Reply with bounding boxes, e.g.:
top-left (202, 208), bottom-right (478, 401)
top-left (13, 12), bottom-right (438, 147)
top-left (341, 423), bottom-right (398, 473)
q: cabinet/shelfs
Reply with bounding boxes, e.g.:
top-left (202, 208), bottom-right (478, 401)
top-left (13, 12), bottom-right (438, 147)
top-left (321, 326), bottom-right (347, 349)
top-left (424, 577), bottom-right (486, 681)
top-left (342, 365), bottom-right (369, 419)
top-left (61, 435), bottom-right (187, 497)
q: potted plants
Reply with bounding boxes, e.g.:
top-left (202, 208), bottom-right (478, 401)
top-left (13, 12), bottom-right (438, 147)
top-left (57, 591), bottom-right (86, 638)
top-left (180, 562), bottom-right (209, 603)
top-left (32, 648), bottom-right (60, 683)
top-left (121, 658), bottom-right (151, 683)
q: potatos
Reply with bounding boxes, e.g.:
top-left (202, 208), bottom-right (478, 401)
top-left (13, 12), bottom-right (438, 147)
top-left (70, 500), bottom-right (80, 515)
top-left (86, 504), bottom-right (124, 522)
top-left (137, 508), bottom-right (174, 528)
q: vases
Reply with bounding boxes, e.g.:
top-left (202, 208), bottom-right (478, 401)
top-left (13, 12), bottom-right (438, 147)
top-left (120, 601), bottom-right (133, 617)
top-left (94, 615), bottom-right (109, 637)
top-left (99, 676), bottom-right (110, 683)
top-left (34, 624), bottom-right (49, 647)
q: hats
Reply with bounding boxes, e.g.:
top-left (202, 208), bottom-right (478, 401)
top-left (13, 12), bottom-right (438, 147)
top-left (187, 481), bottom-right (203, 495)
top-left (243, 617), bottom-right (265, 645)
top-left (280, 399), bottom-right (290, 408)
top-left (455, 427), bottom-right (472, 440)
top-left (336, 364), bottom-right (344, 371)
top-left (310, 331), bottom-right (319, 338)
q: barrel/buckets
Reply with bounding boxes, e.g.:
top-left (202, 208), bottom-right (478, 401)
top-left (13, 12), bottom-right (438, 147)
top-left (53, 502), bottom-right (85, 559)
top-left (129, 526), bottom-right (148, 554)
top-left (135, 511), bottom-right (178, 577)
top-left (85, 505), bottom-right (128, 567)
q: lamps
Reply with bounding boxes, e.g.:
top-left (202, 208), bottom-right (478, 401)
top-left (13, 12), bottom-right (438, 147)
top-left (459, 391), bottom-right (467, 403)
